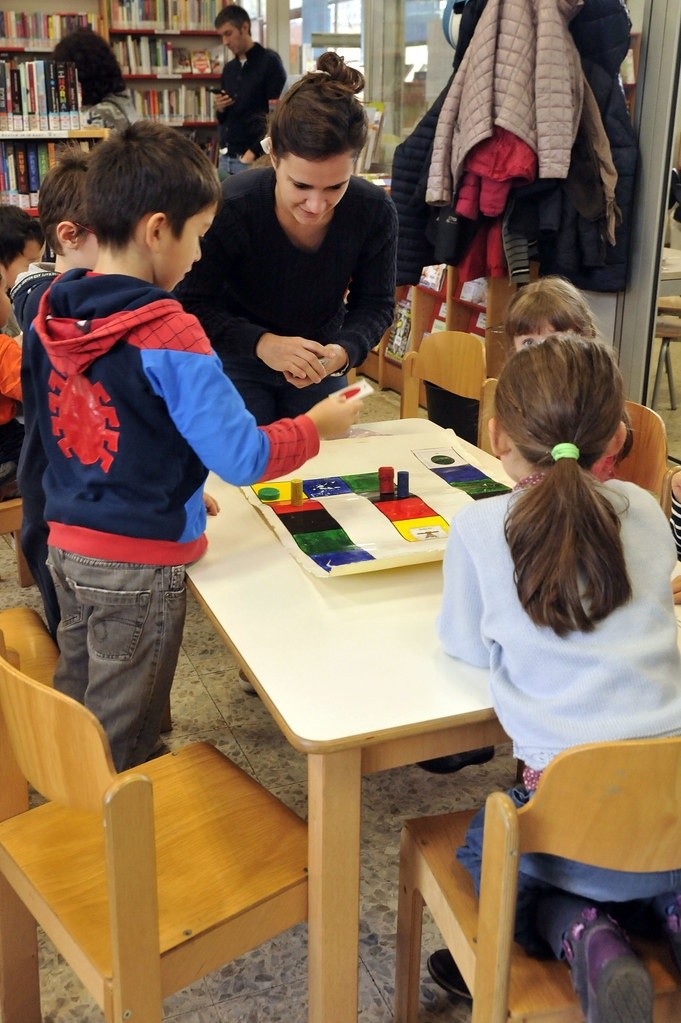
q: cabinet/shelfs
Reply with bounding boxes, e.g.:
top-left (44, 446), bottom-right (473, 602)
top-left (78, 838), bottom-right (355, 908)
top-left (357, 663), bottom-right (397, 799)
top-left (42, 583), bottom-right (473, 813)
top-left (0, 0), bottom-right (233, 218)
top-left (346, 262), bottom-right (540, 413)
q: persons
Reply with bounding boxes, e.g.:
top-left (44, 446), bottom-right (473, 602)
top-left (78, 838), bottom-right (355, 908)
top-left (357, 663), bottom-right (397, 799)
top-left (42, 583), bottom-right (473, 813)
top-left (56, 26), bottom-right (140, 148)
top-left (0, 120), bottom-right (363, 773)
top-left (427, 332), bottom-right (681, 1023)
top-left (508, 276), bottom-right (627, 483)
top-left (174, 53), bottom-right (399, 422)
top-left (214, 5), bottom-right (286, 180)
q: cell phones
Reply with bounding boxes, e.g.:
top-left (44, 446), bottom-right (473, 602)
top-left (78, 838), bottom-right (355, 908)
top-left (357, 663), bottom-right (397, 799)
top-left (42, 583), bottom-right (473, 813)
top-left (212, 88), bottom-right (228, 97)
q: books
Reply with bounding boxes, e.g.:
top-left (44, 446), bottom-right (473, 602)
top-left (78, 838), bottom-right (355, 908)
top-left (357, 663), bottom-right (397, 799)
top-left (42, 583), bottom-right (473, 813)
top-left (0, 10), bottom-right (105, 263)
top-left (107, 0), bottom-right (237, 169)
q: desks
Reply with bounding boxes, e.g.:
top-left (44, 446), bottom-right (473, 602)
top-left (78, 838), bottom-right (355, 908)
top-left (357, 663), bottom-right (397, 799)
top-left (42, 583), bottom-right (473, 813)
top-left (157, 418), bottom-right (520, 1023)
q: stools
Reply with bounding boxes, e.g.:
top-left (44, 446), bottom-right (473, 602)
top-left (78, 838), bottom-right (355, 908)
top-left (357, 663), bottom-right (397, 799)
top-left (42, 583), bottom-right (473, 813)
top-left (651, 315), bottom-right (681, 410)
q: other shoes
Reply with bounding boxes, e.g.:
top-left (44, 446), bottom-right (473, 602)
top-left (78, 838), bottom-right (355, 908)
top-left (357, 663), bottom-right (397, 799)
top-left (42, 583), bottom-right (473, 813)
top-left (564, 918), bottom-right (654, 1023)
top-left (667, 895), bottom-right (681, 970)
top-left (239, 668), bottom-right (255, 692)
top-left (426, 949), bottom-right (473, 1000)
top-left (416, 744), bottom-right (494, 773)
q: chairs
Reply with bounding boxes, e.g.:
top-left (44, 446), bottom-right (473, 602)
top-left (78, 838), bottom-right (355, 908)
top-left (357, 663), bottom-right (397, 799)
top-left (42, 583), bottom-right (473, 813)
top-left (0, 630), bottom-right (307, 1023)
top-left (398, 330), bottom-right (498, 458)
top-left (398, 738), bottom-right (681, 1023)
top-left (612, 401), bottom-right (669, 505)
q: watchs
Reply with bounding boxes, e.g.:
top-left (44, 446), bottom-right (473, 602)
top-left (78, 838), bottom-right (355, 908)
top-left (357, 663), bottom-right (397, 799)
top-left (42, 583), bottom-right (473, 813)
top-left (329, 358), bottom-right (349, 377)
